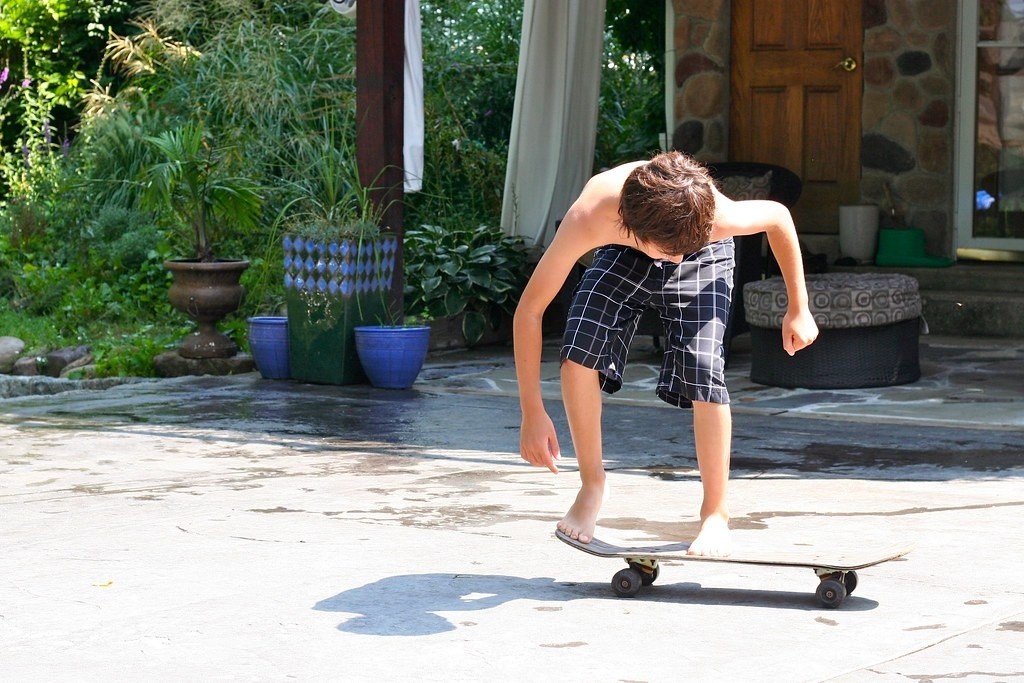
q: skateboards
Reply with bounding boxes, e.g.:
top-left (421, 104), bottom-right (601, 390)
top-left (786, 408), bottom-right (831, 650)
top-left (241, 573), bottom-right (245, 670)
top-left (555, 529), bottom-right (915, 607)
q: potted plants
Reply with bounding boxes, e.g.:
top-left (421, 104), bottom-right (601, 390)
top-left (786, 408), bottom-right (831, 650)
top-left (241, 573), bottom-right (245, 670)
top-left (254, 105), bottom-right (399, 385)
top-left (128, 120), bottom-right (273, 360)
top-left (354, 301), bottom-right (432, 390)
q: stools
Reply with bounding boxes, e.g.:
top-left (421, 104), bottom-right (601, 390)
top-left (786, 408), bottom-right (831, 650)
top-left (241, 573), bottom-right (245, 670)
top-left (743, 271), bottom-right (922, 390)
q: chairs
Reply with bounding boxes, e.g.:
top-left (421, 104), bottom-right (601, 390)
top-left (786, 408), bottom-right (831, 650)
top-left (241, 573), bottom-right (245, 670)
top-left (555, 162), bottom-right (802, 370)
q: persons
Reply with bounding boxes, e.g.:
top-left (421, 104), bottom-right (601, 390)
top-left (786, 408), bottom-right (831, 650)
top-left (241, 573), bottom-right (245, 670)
top-left (512, 150), bottom-right (818, 556)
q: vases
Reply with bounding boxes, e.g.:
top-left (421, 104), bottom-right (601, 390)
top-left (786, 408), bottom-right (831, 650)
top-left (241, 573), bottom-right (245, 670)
top-left (246, 316), bottom-right (290, 380)
